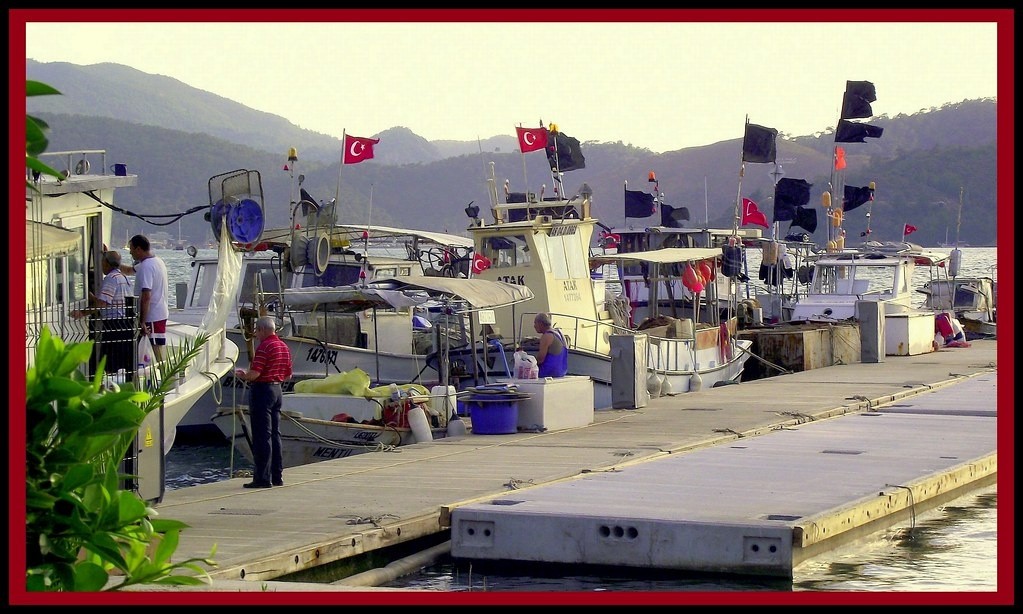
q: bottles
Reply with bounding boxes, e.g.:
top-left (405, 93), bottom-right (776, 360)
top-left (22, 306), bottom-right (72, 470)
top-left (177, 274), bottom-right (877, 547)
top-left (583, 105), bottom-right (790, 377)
top-left (390, 384), bottom-right (401, 402)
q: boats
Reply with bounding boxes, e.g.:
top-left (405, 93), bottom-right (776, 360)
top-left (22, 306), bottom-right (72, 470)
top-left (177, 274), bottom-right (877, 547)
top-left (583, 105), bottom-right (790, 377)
top-left (158, 123), bottom-right (997, 466)
top-left (22, 150), bottom-right (238, 455)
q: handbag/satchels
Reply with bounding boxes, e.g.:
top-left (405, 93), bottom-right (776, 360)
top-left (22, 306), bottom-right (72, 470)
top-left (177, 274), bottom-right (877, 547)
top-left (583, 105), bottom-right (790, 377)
top-left (514, 351), bottom-right (539, 379)
top-left (138, 334), bottom-right (156, 368)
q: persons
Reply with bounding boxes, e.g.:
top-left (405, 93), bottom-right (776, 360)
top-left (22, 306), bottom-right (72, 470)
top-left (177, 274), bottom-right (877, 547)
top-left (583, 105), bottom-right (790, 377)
top-left (235, 316), bottom-right (293, 488)
top-left (534, 313), bottom-right (567, 378)
top-left (68, 251), bottom-right (134, 368)
top-left (104, 234), bottom-right (168, 362)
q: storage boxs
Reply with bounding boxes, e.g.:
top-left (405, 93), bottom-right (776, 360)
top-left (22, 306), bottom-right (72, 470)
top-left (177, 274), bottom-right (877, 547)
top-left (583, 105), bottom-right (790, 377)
top-left (497, 375), bottom-right (594, 431)
top-left (884, 311), bottom-right (935, 355)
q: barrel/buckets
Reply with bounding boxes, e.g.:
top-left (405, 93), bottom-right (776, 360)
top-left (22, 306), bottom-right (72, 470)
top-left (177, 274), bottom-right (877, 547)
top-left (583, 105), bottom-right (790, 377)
top-left (469, 392), bottom-right (519, 434)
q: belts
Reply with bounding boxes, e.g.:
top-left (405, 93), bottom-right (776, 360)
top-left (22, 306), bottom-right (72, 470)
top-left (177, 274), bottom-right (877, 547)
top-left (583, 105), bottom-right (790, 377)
top-left (252, 381), bottom-right (279, 385)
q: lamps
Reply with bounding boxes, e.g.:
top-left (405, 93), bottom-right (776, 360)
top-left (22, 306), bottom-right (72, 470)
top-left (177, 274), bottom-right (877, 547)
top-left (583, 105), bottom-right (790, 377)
top-left (464, 205), bottom-right (479, 219)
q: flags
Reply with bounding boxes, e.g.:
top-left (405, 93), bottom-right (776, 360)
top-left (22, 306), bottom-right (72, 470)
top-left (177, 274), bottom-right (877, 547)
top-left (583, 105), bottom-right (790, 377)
top-left (472, 254), bottom-right (489, 274)
top-left (544, 131), bottom-right (585, 175)
top-left (343, 135), bottom-right (379, 164)
top-left (660, 203), bottom-right (690, 230)
top-left (836, 80), bottom-right (884, 143)
top-left (743, 124), bottom-right (777, 164)
top-left (624, 190), bottom-right (655, 218)
top-left (300, 188), bottom-right (320, 214)
top-left (905, 224), bottom-right (917, 236)
top-left (740, 198), bottom-right (768, 229)
top-left (517, 128), bottom-right (548, 153)
top-left (844, 185), bottom-right (870, 212)
top-left (775, 178), bottom-right (817, 233)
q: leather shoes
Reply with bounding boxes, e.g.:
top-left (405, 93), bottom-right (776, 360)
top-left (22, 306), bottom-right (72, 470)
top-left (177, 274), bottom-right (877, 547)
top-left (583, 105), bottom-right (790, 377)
top-left (244, 482), bottom-right (273, 488)
top-left (273, 478), bottom-right (284, 486)
